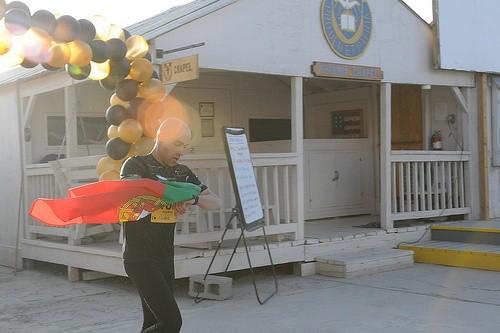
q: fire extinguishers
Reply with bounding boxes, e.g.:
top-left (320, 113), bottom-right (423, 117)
top-left (431, 130), bottom-right (442, 167)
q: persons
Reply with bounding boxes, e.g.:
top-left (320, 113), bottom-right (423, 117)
top-left (122, 117), bottom-right (223, 333)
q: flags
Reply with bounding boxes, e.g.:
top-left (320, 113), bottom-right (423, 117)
top-left (30, 179), bottom-right (201, 226)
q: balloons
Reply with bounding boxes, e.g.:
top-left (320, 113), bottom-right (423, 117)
top-left (0, 0), bottom-right (183, 181)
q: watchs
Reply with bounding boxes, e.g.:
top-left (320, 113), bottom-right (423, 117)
top-left (194, 196), bottom-right (199, 206)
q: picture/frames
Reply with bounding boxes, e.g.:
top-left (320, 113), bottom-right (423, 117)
top-left (44, 111), bottom-right (108, 147)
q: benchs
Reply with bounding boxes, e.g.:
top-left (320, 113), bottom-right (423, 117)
top-left (178, 205), bottom-right (281, 249)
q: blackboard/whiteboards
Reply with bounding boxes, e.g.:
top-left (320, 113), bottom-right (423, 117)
top-left (221, 126), bottom-right (263, 227)
